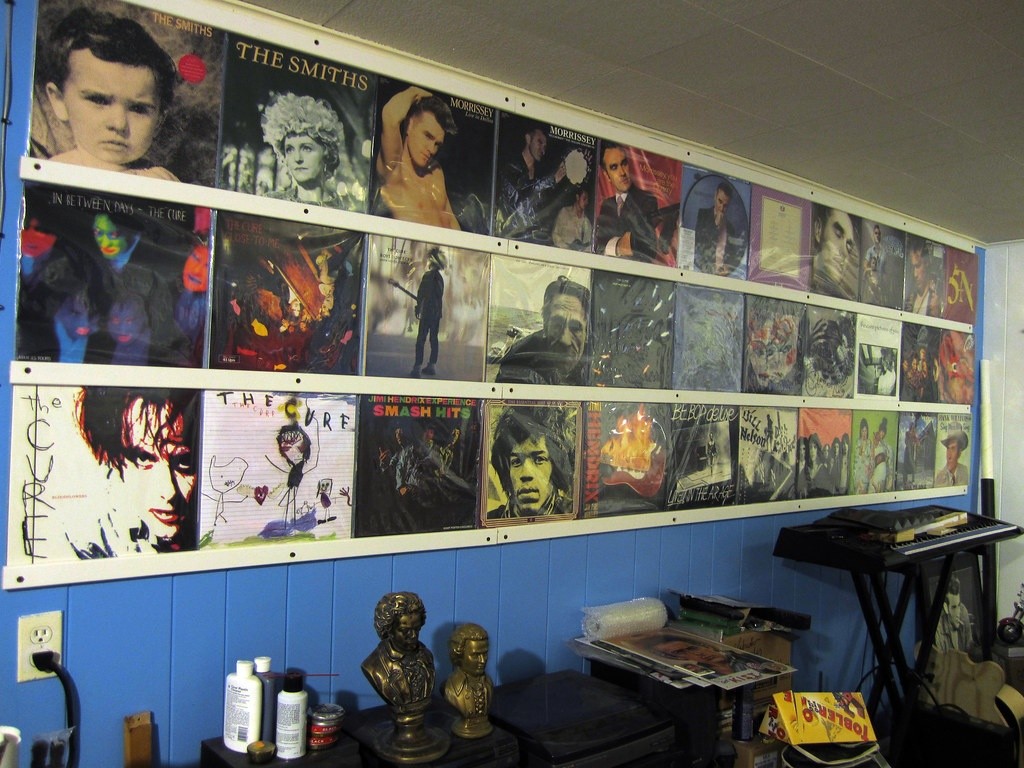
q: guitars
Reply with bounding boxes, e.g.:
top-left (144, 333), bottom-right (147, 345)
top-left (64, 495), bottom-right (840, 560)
top-left (387, 278), bottom-right (418, 300)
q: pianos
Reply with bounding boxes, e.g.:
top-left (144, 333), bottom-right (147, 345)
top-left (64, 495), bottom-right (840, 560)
top-left (771, 503), bottom-right (1024, 574)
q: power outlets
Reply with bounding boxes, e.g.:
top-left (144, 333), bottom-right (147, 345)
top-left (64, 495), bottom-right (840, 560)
top-left (17, 610), bottom-right (63, 682)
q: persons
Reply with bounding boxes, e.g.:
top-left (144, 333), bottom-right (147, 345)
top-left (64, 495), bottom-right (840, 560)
top-left (634, 634), bottom-right (781, 680)
top-left (439, 623), bottom-right (494, 726)
top-left (361, 591), bottom-right (437, 715)
top-left (39, 9), bottom-right (971, 562)
top-left (935, 575), bottom-right (972, 653)
